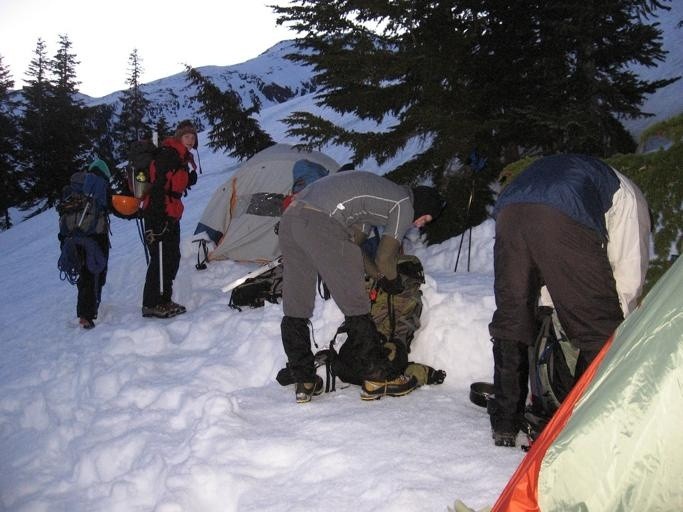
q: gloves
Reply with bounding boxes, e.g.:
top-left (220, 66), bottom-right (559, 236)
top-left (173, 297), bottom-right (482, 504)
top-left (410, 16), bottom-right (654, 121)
top-left (188, 170), bottom-right (197, 185)
top-left (382, 273), bottom-right (405, 296)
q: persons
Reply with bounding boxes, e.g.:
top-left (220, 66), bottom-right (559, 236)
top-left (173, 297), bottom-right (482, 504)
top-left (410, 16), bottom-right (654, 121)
top-left (142, 121), bottom-right (198, 319)
top-left (58, 159), bottom-right (113, 329)
top-left (129, 131), bottom-right (156, 175)
top-left (490, 153), bottom-right (651, 446)
top-left (279, 173), bottom-right (442, 404)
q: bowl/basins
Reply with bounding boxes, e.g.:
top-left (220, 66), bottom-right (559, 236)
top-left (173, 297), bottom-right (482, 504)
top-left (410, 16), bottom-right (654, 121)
top-left (469, 382), bottom-right (495, 408)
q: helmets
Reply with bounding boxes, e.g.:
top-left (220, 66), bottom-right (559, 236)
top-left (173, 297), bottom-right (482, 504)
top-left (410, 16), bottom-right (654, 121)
top-left (111, 194), bottom-right (141, 215)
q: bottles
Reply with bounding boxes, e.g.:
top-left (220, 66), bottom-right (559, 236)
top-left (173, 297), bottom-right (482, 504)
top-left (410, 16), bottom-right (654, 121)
top-left (134, 171), bottom-right (147, 198)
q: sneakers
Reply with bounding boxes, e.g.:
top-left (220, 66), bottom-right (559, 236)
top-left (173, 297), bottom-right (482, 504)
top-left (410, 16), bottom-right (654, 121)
top-left (79, 313), bottom-right (95, 328)
top-left (361, 374), bottom-right (417, 400)
top-left (295, 375), bottom-right (324, 402)
top-left (143, 301), bottom-right (186, 318)
top-left (489, 424), bottom-right (518, 446)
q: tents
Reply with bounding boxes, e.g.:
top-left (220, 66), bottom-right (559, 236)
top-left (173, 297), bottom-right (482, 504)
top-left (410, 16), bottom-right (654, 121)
top-left (195, 144), bottom-right (341, 262)
top-left (489, 257), bottom-right (683, 511)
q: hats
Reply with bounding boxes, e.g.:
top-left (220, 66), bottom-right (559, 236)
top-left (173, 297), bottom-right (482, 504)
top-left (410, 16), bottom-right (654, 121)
top-left (176, 119), bottom-right (198, 150)
top-left (89, 160), bottom-right (111, 178)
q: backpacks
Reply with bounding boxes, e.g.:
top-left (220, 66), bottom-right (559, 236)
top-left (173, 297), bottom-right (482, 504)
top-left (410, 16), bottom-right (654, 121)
top-left (57, 191), bottom-right (95, 236)
top-left (528, 305), bottom-right (591, 416)
top-left (328, 254), bottom-right (425, 386)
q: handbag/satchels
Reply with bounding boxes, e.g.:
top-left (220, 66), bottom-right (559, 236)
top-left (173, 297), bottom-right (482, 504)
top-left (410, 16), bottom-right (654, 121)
top-left (229, 275), bottom-right (271, 308)
